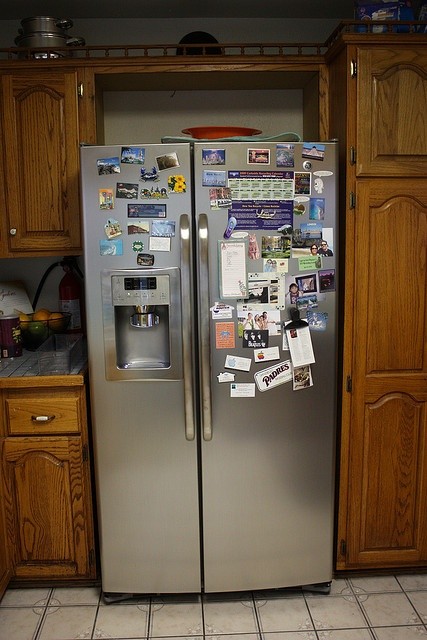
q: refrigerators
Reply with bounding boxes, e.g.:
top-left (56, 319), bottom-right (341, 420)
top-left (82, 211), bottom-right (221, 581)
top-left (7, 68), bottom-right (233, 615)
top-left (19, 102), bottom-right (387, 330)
top-left (79, 142), bottom-right (340, 592)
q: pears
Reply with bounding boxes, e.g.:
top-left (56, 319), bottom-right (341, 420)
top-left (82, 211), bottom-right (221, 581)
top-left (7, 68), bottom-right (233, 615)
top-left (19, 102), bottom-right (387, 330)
top-left (28, 322), bottom-right (46, 338)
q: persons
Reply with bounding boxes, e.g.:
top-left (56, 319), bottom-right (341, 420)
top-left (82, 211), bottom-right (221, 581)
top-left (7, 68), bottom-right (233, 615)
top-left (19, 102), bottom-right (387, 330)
top-left (242, 312), bottom-right (269, 331)
top-left (310, 244), bottom-right (322, 257)
top-left (317, 240), bottom-right (333, 257)
top-left (244, 332), bottom-right (267, 348)
top-left (263, 259), bottom-right (278, 272)
top-left (285, 283), bottom-right (302, 304)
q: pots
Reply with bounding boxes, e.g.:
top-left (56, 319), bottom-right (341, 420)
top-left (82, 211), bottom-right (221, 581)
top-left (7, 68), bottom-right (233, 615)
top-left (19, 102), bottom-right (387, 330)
top-left (17, 16), bottom-right (75, 34)
top-left (14, 32), bottom-right (86, 57)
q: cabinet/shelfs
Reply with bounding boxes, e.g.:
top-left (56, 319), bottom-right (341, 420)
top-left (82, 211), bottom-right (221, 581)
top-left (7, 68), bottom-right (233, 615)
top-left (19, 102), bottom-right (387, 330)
top-left (5, 379), bottom-right (91, 587)
top-left (339, 32), bottom-right (427, 568)
top-left (1, 65), bottom-right (81, 257)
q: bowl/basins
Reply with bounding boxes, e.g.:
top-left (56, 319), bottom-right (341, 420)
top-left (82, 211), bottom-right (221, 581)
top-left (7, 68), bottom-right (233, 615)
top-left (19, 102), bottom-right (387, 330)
top-left (181, 125), bottom-right (262, 139)
top-left (19, 312), bottom-right (72, 351)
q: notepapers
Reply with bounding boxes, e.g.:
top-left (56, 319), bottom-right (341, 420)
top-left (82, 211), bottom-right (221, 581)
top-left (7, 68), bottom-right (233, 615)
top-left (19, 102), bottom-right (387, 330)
top-left (215, 322), bottom-right (235, 349)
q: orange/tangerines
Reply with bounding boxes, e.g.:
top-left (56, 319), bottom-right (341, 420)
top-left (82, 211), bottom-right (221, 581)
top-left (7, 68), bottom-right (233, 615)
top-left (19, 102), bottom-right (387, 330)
top-left (32, 308), bottom-right (51, 326)
top-left (48, 312), bottom-right (65, 330)
top-left (19, 314), bottom-right (30, 331)
